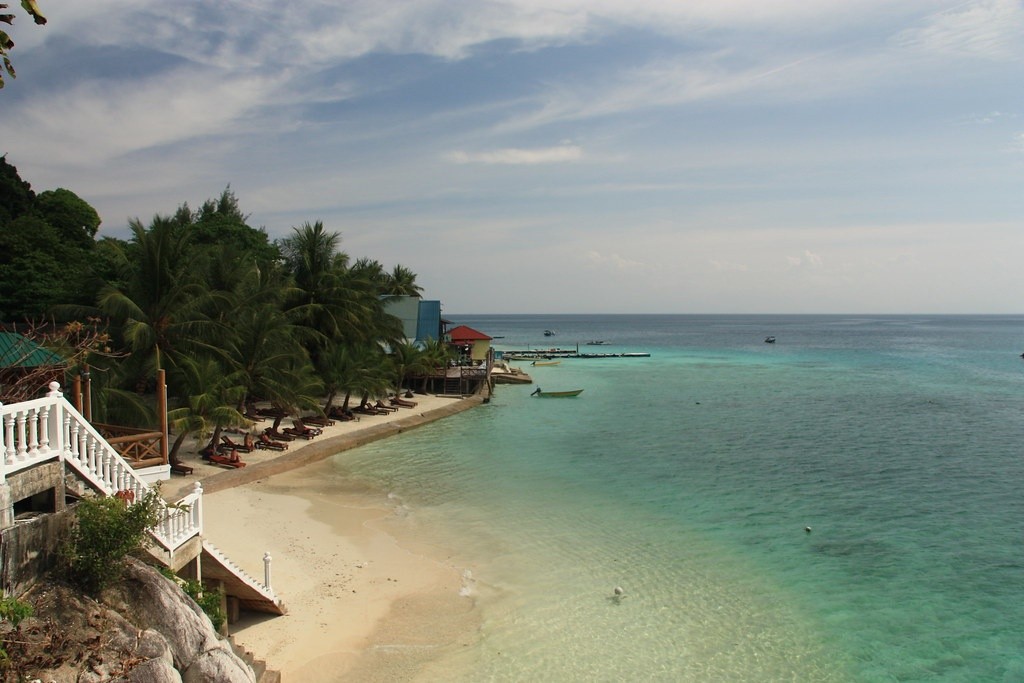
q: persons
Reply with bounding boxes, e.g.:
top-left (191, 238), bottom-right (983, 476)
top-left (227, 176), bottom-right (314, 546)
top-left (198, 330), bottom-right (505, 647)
top-left (211, 442), bottom-right (216, 455)
top-left (336, 407), bottom-right (351, 419)
top-left (218, 443), bottom-right (228, 456)
top-left (261, 431), bottom-right (287, 446)
top-left (230, 447), bottom-right (240, 463)
top-left (244, 432), bottom-right (254, 452)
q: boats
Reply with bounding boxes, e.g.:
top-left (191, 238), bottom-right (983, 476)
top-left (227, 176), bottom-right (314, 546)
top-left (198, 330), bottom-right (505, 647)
top-left (537, 389), bottom-right (585, 398)
top-left (586, 340), bottom-right (611, 346)
top-left (543, 329), bottom-right (556, 337)
top-left (532, 360), bottom-right (563, 368)
top-left (764, 335), bottom-right (776, 343)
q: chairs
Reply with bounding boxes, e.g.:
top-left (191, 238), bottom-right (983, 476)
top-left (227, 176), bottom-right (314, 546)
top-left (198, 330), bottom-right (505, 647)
top-left (358, 406), bottom-right (379, 415)
top-left (292, 420), bottom-right (323, 436)
top-left (221, 435), bottom-right (254, 453)
top-left (209, 455), bottom-right (246, 468)
top-left (281, 428), bottom-right (315, 440)
top-left (365, 403), bottom-right (392, 415)
top-left (171, 464), bottom-right (194, 476)
top-left (243, 403), bottom-right (289, 422)
top-left (374, 400), bottom-right (399, 412)
top-left (257, 434), bottom-right (289, 451)
top-left (265, 427), bottom-right (297, 441)
top-left (389, 397), bottom-right (417, 409)
top-left (300, 406), bottom-right (354, 428)
